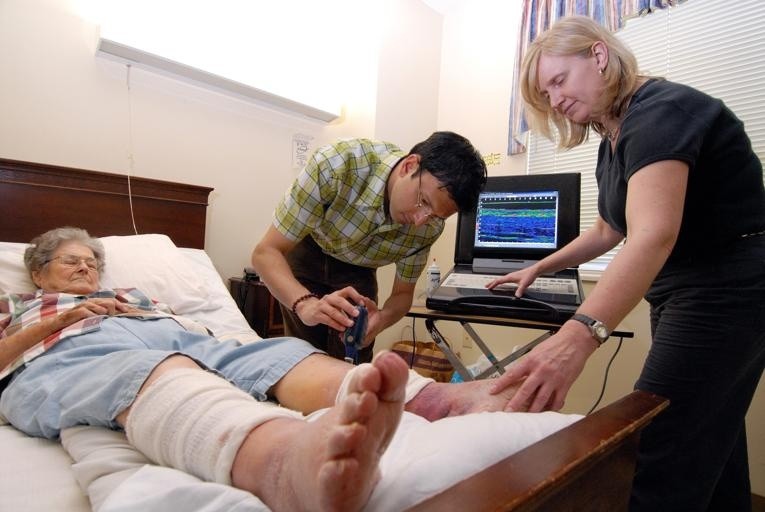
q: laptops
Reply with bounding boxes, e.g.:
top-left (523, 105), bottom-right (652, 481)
top-left (426, 171), bottom-right (586, 321)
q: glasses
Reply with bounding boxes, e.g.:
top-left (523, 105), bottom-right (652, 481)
top-left (42, 254), bottom-right (101, 274)
top-left (416, 168), bottom-right (449, 223)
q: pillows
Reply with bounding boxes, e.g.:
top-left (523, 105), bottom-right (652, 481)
top-left (0, 232), bottom-right (222, 317)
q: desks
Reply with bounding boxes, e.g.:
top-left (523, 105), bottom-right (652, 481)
top-left (402, 306), bottom-right (634, 382)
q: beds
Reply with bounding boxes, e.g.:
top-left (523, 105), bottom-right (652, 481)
top-left (1, 157), bottom-right (672, 512)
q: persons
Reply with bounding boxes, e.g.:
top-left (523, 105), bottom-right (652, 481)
top-left (486, 16), bottom-right (764, 510)
top-left (0, 225), bottom-right (562, 512)
top-left (251, 130), bottom-right (489, 363)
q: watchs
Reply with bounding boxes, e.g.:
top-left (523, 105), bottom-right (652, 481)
top-left (571, 314), bottom-right (611, 347)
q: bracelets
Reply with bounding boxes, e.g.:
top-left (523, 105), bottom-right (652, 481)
top-left (291, 292), bottom-right (322, 314)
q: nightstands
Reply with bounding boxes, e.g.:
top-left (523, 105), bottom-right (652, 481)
top-left (229, 276), bottom-right (287, 338)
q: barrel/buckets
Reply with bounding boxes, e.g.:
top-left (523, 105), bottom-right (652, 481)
top-left (391, 325), bottom-right (461, 382)
